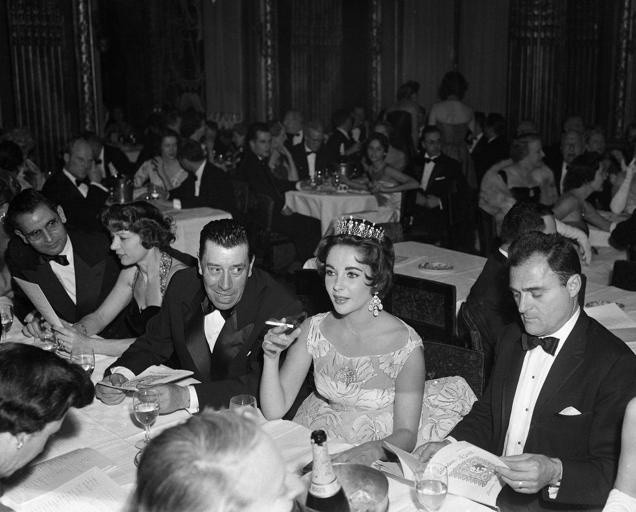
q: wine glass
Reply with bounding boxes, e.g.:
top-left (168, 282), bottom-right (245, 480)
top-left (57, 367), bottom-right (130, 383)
top-left (0, 303), bottom-right (14, 343)
top-left (300, 169), bottom-right (357, 195)
top-left (70, 339), bottom-right (94, 381)
top-left (412, 462), bottom-right (452, 512)
top-left (149, 179), bottom-right (160, 200)
top-left (131, 387), bottom-right (160, 452)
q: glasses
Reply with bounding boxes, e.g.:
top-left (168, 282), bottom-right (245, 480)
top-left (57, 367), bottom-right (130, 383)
top-left (25, 214), bottom-right (60, 240)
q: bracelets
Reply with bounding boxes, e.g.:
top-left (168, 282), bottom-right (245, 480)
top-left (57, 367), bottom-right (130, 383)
top-left (73, 323), bottom-right (87, 335)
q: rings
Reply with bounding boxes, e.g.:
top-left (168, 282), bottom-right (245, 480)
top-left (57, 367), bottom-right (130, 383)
top-left (518, 481), bottom-right (524, 489)
top-left (269, 334), bottom-right (276, 341)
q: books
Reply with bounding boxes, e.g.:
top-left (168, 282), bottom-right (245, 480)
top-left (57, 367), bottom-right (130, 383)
top-left (370, 437), bottom-right (511, 512)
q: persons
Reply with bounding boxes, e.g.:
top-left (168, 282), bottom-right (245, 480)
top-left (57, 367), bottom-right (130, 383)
top-left (260, 216), bottom-right (428, 474)
top-left (3, 188), bottom-right (133, 338)
top-left (123, 406), bottom-right (305, 511)
top-left (464, 201), bottom-right (556, 369)
top-left (96, 217), bottom-right (304, 415)
top-left (410, 229), bottom-right (636, 512)
top-left (0, 342), bottom-right (94, 510)
top-left (329, 71), bottom-right (636, 245)
top-left (1, 105), bottom-right (325, 274)
top-left (41, 202), bottom-right (189, 357)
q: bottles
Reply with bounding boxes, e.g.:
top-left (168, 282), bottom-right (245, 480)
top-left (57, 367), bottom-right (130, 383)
top-left (305, 427), bottom-right (350, 510)
top-left (108, 161), bottom-right (123, 180)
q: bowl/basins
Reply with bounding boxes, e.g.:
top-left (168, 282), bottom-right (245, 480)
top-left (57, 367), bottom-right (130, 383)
top-left (294, 462), bottom-right (391, 512)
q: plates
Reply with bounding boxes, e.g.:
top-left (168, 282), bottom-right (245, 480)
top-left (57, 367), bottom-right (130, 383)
top-left (584, 300), bottom-right (625, 313)
top-left (417, 261), bottom-right (456, 274)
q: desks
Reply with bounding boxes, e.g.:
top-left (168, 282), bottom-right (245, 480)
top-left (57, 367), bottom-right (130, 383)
top-left (101, 185), bottom-right (233, 275)
top-left (304, 240), bottom-right (635, 354)
top-left (0, 308), bottom-right (494, 511)
top-left (282, 179), bottom-right (403, 239)
top-left (562, 207), bottom-right (636, 275)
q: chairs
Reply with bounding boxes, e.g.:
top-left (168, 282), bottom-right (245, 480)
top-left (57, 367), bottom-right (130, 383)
top-left (382, 266), bottom-right (457, 338)
top-left (409, 341), bottom-right (485, 406)
top-left (436, 186), bottom-right (499, 254)
top-left (227, 175), bottom-right (302, 280)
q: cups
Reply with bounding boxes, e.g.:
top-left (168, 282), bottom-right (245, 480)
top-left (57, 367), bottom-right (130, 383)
top-left (228, 396), bottom-right (262, 416)
top-left (30, 330), bottom-right (54, 352)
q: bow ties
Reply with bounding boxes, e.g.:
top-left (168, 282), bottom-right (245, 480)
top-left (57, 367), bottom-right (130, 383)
top-left (425, 157), bottom-right (439, 164)
top-left (521, 333), bottom-right (558, 356)
top-left (37, 254), bottom-right (68, 266)
top-left (306, 151), bottom-right (317, 155)
top-left (75, 178), bottom-right (89, 186)
top-left (200, 295), bottom-right (233, 320)
